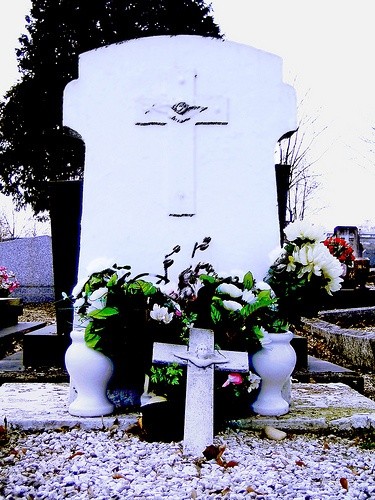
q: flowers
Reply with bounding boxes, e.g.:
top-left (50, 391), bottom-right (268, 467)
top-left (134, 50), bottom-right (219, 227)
top-left (267, 235), bottom-right (358, 295)
top-left (61, 270), bottom-right (284, 394)
top-left (0, 265), bottom-right (23, 291)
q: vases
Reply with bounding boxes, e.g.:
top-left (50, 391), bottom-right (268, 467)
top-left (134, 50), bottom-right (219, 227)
top-left (65, 320), bottom-right (115, 421)
top-left (0, 286), bottom-right (15, 298)
top-left (250, 324), bottom-right (298, 420)
